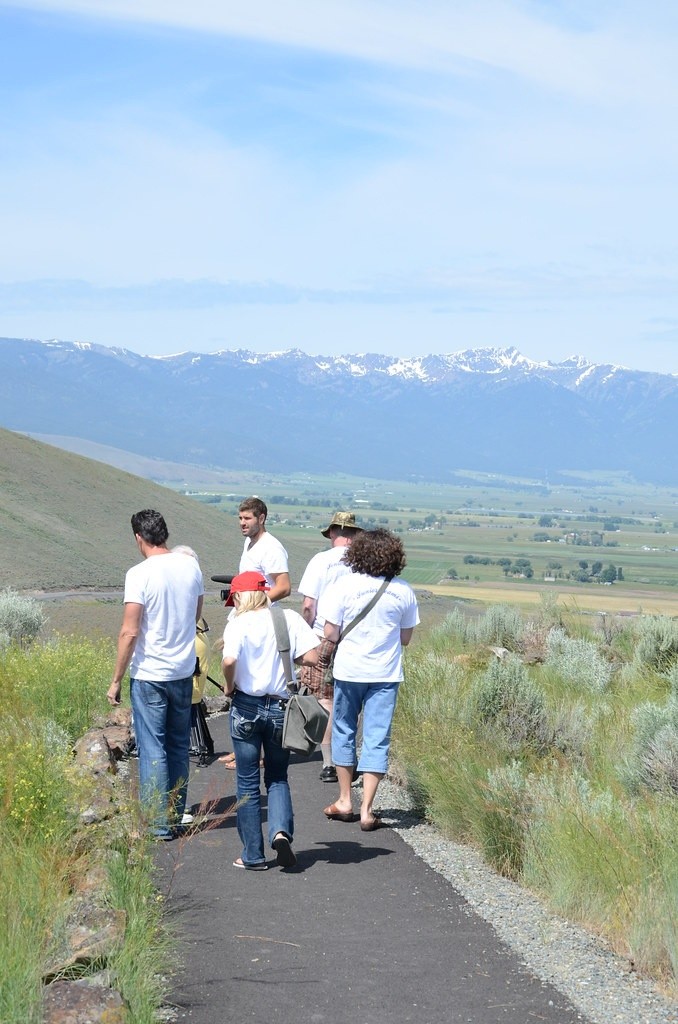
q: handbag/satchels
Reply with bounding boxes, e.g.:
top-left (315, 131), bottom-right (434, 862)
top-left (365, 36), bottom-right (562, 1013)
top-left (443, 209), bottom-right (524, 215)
top-left (281, 692), bottom-right (329, 756)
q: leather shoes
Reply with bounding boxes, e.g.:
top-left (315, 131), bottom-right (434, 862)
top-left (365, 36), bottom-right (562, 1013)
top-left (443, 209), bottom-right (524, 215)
top-left (319, 765), bottom-right (337, 782)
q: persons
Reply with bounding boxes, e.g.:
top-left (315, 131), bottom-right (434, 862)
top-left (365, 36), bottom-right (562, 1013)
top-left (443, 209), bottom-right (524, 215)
top-left (107, 509), bottom-right (203, 842)
top-left (295, 513), bottom-right (368, 782)
top-left (321, 529), bottom-right (421, 831)
top-left (191, 619), bottom-right (209, 717)
top-left (215, 497), bottom-right (290, 771)
top-left (224, 571), bottom-right (321, 870)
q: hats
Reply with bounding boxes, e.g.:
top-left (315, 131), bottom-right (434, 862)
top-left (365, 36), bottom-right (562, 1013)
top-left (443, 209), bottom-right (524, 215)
top-left (321, 512), bottom-right (366, 539)
top-left (223, 570), bottom-right (270, 606)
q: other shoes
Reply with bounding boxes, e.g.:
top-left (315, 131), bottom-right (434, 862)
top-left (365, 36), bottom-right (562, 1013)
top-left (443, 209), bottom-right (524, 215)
top-left (145, 823), bottom-right (175, 842)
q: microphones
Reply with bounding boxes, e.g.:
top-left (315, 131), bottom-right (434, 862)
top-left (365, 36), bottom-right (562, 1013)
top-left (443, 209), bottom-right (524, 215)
top-left (211, 575), bottom-right (235, 584)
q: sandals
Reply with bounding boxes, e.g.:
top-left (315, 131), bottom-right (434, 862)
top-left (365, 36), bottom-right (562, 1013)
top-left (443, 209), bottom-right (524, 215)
top-left (322, 799), bottom-right (353, 822)
top-left (360, 813), bottom-right (378, 831)
top-left (225, 759), bottom-right (238, 770)
top-left (232, 858), bottom-right (268, 870)
top-left (219, 752), bottom-right (235, 763)
top-left (274, 833), bottom-right (297, 867)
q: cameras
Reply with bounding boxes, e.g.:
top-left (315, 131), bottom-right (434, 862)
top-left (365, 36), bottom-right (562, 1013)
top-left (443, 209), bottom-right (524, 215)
top-left (221, 589), bottom-right (231, 601)
